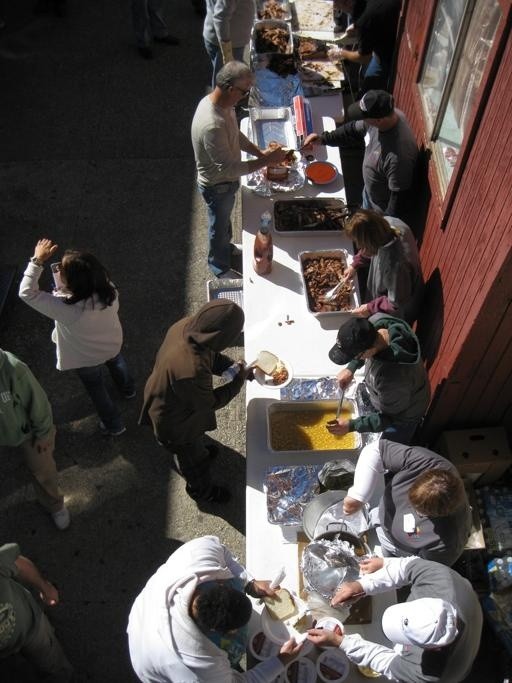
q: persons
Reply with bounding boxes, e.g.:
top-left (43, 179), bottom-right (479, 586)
top-left (136, 297), bottom-right (261, 502)
top-left (1, 347), bottom-right (72, 532)
top-left (306, 553), bottom-right (483, 682)
top-left (324, 313), bottom-right (432, 447)
top-left (326, 0), bottom-right (401, 102)
top-left (1, 541), bottom-right (76, 681)
top-left (125, 534), bottom-right (306, 682)
top-left (340, 207), bottom-right (427, 328)
top-left (202, 0), bottom-right (258, 121)
top-left (191, 59), bottom-right (288, 279)
top-left (303, 88), bottom-right (420, 217)
top-left (341, 438), bottom-right (472, 566)
top-left (17, 236), bottom-right (136, 435)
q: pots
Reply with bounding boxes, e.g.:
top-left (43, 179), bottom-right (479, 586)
top-left (302, 488), bottom-right (372, 608)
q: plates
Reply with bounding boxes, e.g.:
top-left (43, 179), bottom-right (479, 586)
top-left (250, 353), bottom-right (293, 389)
top-left (306, 161), bottom-right (338, 185)
top-left (260, 596), bottom-right (313, 647)
top-left (269, 146), bottom-right (302, 169)
top-left (358, 666), bottom-right (383, 678)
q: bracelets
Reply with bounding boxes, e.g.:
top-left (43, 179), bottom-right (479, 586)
top-left (28, 256), bottom-right (45, 266)
top-left (246, 578), bottom-right (256, 593)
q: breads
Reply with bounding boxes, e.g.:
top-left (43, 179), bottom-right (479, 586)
top-left (264, 589), bottom-right (295, 620)
top-left (257, 351), bottom-right (279, 374)
top-left (266, 167), bottom-right (289, 182)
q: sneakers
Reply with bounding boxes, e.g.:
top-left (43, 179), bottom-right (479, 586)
top-left (186, 479), bottom-right (231, 504)
top-left (205, 444), bottom-right (219, 459)
top-left (51, 501), bottom-right (70, 530)
top-left (123, 375), bottom-right (136, 399)
top-left (99, 419), bottom-right (126, 436)
top-left (137, 33), bottom-right (179, 60)
top-left (210, 244), bottom-right (242, 278)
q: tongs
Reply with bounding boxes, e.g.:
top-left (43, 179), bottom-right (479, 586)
top-left (324, 271), bottom-right (352, 303)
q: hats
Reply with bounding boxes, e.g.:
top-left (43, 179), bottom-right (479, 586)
top-left (381, 597), bottom-right (458, 649)
top-left (328, 317), bottom-right (373, 365)
top-left (348, 88), bottom-right (395, 120)
top-left (182, 298), bottom-right (245, 348)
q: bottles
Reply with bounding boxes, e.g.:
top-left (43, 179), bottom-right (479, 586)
top-left (251, 211), bottom-right (273, 276)
